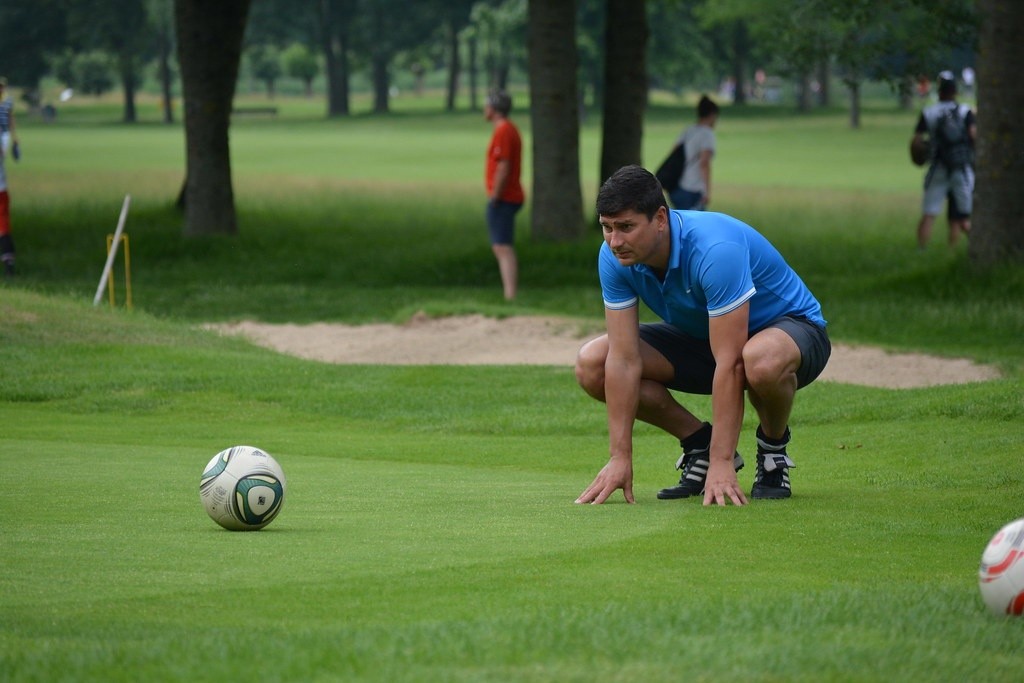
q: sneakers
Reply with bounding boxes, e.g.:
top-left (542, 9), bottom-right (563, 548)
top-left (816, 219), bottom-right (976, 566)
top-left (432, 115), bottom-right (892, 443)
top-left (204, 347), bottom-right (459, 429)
top-left (657, 422), bottom-right (745, 499)
top-left (751, 422), bottom-right (796, 499)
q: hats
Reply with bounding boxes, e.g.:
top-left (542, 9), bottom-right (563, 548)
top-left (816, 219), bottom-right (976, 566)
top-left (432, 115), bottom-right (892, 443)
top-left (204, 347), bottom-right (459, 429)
top-left (939, 70), bottom-right (953, 80)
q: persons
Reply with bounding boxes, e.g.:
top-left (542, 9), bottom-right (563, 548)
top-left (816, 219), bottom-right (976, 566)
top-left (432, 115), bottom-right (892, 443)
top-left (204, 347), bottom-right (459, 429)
top-left (574, 164), bottom-right (832, 507)
top-left (911, 80), bottom-right (980, 249)
top-left (0, 79), bottom-right (20, 271)
top-left (655, 95), bottom-right (721, 212)
top-left (484, 86), bottom-right (525, 300)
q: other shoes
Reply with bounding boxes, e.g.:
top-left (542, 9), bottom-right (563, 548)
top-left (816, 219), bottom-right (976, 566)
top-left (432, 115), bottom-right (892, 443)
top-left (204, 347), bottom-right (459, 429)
top-left (4, 260), bottom-right (15, 280)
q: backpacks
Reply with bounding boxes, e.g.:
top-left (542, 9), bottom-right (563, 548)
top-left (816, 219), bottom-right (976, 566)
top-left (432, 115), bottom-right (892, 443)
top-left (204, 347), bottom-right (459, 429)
top-left (934, 110), bottom-right (974, 162)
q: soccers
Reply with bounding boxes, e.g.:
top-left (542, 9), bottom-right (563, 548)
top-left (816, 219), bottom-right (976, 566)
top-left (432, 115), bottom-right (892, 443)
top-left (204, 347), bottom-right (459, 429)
top-left (199, 445), bottom-right (289, 535)
top-left (979, 518), bottom-right (1024, 616)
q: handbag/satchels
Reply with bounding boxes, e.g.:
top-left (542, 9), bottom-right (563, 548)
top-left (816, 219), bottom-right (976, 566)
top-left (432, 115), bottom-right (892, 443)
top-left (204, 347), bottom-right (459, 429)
top-left (656, 144), bottom-right (685, 193)
